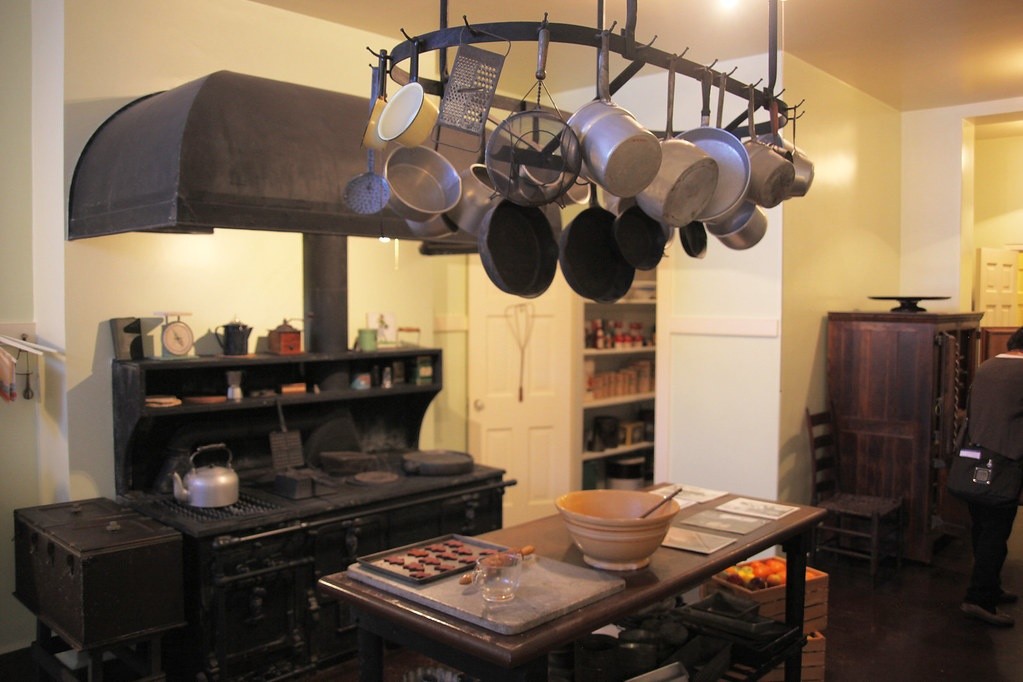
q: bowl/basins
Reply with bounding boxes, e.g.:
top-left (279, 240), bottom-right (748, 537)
top-left (555, 490), bottom-right (681, 570)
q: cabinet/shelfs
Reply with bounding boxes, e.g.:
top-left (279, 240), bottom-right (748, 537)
top-left (197, 471), bottom-right (508, 682)
top-left (825, 311), bottom-right (985, 561)
top-left (578, 298), bottom-right (656, 490)
top-left (113, 339), bottom-right (441, 497)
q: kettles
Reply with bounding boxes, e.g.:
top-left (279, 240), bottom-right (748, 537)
top-left (166, 444), bottom-right (240, 508)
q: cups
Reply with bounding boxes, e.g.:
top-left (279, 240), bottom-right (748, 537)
top-left (604, 454), bottom-right (647, 491)
top-left (616, 628), bottom-right (664, 679)
top-left (358, 329), bottom-right (378, 354)
top-left (349, 372), bottom-right (372, 389)
top-left (470, 551), bottom-right (523, 603)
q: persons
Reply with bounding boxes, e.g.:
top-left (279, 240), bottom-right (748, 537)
top-left (958, 327), bottom-right (1023, 628)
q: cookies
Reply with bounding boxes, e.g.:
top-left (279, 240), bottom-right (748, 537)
top-left (383, 540), bottom-right (498, 580)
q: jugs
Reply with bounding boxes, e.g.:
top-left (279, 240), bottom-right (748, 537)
top-left (215, 319), bottom-right (253, 356)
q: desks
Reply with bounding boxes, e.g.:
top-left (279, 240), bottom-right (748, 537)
top-left (319, 481), bottom-right (825, 682)
top-left (27, 618), bottom-right (188, 682)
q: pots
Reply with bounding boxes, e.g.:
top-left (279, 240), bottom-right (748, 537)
top-left (358, 27), bottom-right (815, 303)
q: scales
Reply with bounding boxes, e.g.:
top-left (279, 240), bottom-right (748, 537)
top-left (153, 312), bottom-right (200, 360)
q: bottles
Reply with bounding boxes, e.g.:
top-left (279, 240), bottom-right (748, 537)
top-left (583, 317), bottom-right (656, 350)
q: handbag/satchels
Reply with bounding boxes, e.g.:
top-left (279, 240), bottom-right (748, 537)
top-left (947, 443), bottom-right (1023, 509)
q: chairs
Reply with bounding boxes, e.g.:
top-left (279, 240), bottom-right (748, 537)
top-left (805, 408), bottom-right (902, 578)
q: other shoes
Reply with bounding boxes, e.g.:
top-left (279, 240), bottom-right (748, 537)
top-left (997, 588), bottom-right (1019, 605)
top-left (960, 602), bottom-right (1015, 627)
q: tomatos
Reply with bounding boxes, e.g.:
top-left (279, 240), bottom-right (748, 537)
top-left (717, 560), bottom-right (818, 591)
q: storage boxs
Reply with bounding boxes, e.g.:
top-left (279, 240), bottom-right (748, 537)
top-left (762, 632), bottom-right (826, 682)
top-left (12, 496), bottom-right (183, 646)
top-left (710, 557), bottom-right (830, 633)
